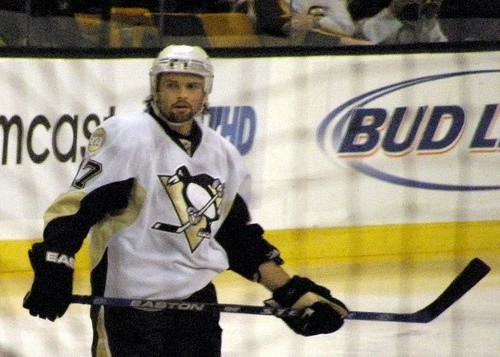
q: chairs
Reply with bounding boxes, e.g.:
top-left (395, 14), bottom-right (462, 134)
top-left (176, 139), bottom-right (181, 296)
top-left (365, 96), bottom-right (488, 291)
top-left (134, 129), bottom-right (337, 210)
top-left (0, 6), bottom-right (499, 52)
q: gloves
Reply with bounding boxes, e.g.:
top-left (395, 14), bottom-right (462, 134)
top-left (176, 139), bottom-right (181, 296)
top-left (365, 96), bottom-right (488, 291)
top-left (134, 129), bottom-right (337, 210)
top-left (22, 241), bottom-right (75, 322)
top-left (264, 275), bottom-right (350, 336)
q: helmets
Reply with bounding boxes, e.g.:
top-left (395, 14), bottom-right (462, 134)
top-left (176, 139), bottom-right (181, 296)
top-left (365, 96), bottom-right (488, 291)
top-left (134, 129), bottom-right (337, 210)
top-left (148, 43), bottom-right (214, 99)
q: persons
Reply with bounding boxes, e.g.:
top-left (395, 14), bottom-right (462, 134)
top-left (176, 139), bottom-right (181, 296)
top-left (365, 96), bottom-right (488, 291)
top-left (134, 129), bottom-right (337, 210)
top-left (22, 45), bottom-right (351, 357)
top-left (238, 0), bottom-right (451, 46)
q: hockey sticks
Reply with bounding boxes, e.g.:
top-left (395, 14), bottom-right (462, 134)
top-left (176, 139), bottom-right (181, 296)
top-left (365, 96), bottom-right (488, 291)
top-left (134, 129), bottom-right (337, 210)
top-left (22, 256), bottom-right (491, 325)
